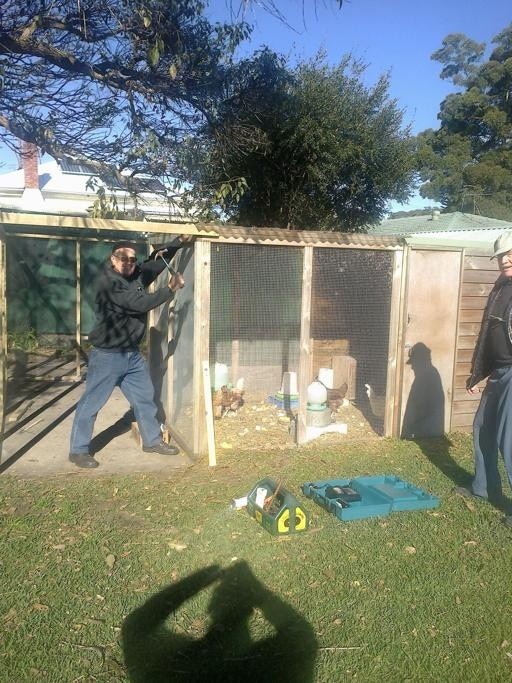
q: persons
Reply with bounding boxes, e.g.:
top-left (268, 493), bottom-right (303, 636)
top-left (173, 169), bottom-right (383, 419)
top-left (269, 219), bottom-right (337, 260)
top-left (454, 231), bottom-right (512, 528)
top-left (67, 235), bottom-right (191, 469)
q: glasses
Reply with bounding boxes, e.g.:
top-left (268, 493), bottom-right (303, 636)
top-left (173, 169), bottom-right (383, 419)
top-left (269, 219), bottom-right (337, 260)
top-left (115, 255), bottom-right (137, 263)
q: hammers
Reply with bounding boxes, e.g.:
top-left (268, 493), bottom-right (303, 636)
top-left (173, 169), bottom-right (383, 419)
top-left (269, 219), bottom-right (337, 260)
top-left (154, 248), bottom-right (184, 290)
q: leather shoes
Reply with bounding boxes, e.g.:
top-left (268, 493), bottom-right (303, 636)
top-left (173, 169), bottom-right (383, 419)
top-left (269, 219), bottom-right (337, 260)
top-left (143, 442), bottom-right (179, 455)
top-left (69, 451), bottom-right (98, 468)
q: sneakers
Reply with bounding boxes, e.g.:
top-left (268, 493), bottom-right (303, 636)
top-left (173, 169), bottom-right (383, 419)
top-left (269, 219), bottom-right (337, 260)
top-left (503, 514), bottom-right (512, 526)
top-left (454, 487), bottom-right (475, 497)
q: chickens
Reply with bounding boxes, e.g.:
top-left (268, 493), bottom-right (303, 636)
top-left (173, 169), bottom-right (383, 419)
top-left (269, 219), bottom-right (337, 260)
top-left (211, 389), bottom-right (223, 418)
top-left (311, 375), bottom-right (348, 418)
top-left (364, 383), bottom-right (385, 417)
top-left (220, 378), bottom-right (245, 419)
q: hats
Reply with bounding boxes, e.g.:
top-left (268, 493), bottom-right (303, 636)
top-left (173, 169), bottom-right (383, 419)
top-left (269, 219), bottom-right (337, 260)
top-left (112, 241), bottom-right (136, 254)
top-left (490, 233), bottom-right (512, 260)
